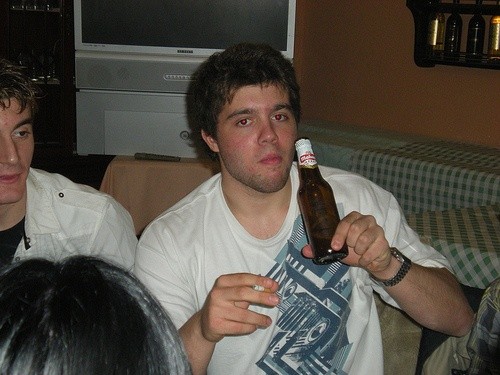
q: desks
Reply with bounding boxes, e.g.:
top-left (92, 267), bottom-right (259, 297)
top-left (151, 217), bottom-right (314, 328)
top-left (98, 155), bottom-right (222, 236)
top-left (294, 124), bottom-right (500, 289)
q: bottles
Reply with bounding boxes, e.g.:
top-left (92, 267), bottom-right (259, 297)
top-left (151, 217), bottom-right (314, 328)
top-left (444, 0), bottom-right (463, 59)
top-left (486, 0), bottom-right (500, 63)
top-left (465, 0), bottom-right (485, 61)
top-left (294, 137), bottom-right (349, 265)
top-left (424, 0), bottom-right (446, 60)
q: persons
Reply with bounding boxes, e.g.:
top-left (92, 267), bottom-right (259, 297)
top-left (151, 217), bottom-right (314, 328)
top-left (0, 59), bottom-right (139, 269)
top-left (0, 254), bottom-right (192, 375)
top-left (135, 41), bottom-right (474, 375)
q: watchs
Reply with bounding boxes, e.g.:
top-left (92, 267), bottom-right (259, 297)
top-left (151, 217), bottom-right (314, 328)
top-left (369, 247), bottom-right (411, 286)
